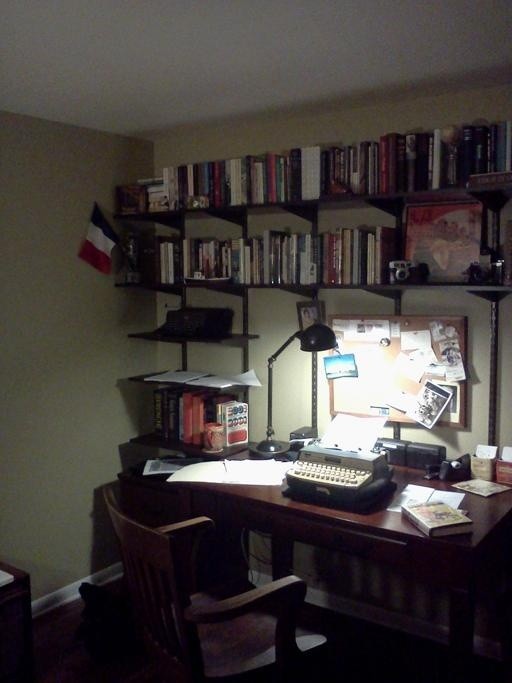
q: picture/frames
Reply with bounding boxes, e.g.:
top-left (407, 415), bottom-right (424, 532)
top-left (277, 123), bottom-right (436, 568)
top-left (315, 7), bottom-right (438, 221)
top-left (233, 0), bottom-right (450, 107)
top-left (296, 302), bottom-right (324, 332)
top-left (401, 201), bottom-right (484, 287)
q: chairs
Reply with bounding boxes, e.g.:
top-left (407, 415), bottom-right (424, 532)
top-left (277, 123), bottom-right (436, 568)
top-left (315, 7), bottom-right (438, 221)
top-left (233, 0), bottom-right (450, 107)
top-left (103, 484), bottom-right (330, 682)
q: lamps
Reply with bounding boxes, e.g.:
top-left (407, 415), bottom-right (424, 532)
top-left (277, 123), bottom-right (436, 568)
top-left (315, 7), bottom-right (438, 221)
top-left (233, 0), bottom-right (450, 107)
top-left (247, 323), bottom-right (338, 459)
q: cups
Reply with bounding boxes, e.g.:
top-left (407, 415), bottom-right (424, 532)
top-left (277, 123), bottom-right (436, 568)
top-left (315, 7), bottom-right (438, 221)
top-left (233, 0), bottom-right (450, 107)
top-left (204, 422), bottom-right (225, 451)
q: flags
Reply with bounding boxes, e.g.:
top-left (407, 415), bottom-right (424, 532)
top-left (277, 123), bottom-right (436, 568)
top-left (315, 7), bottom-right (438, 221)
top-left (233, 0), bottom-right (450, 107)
top-left (78, 201), bottom-right (119, 275)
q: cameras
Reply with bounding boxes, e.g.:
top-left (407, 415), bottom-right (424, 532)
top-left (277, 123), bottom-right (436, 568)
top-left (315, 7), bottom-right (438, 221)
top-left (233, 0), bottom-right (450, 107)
top-left (388, 261), bottom-right (429, 286)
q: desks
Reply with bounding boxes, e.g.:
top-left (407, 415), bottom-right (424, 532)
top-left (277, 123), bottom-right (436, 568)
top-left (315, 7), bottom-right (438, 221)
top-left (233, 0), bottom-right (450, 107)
top-left (115, 440), bottom-right (511, 682)
top-left (0, 563), bottom-right (35, 683)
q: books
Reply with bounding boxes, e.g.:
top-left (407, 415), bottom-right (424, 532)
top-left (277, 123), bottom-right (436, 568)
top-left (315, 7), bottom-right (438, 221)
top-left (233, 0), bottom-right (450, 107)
top-left (154, 386), bottom-right (249, 448)
top-left (140, 119), bottom-right (511, 215)
top-left (161, 226), bottom-right (400, 285)
top-left (401, 500), bottom-right (473, 538)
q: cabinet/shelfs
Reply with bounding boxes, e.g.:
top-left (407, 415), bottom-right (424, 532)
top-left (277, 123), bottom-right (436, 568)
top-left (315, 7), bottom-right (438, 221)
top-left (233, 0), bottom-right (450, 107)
top-left (110, 172), bottom-right (510, 459)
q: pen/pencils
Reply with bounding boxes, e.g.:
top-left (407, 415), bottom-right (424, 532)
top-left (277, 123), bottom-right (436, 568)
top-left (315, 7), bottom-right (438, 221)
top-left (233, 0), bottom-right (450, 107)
top-left (224, 458), bottom-right (228, 471)
